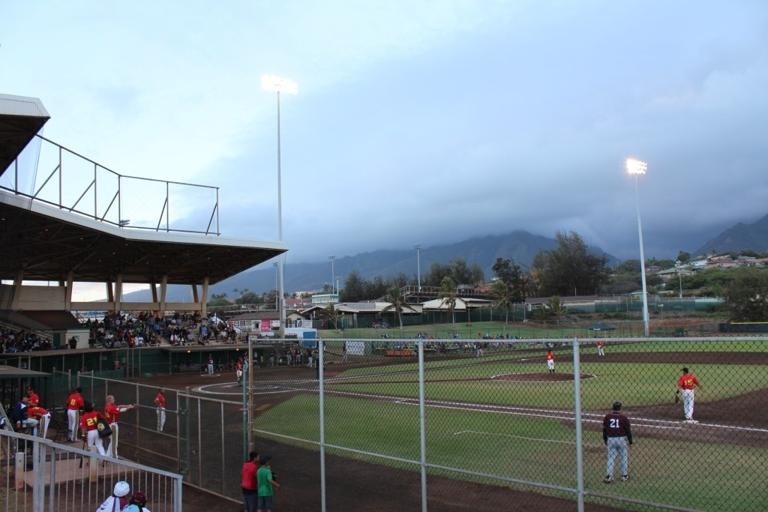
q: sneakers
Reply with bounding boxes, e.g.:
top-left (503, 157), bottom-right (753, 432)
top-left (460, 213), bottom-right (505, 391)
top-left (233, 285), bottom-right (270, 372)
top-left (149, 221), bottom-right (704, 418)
top-left (604, 476), bottom-right (611, 483)
top-left (621, 475), bottom-right (629, 481)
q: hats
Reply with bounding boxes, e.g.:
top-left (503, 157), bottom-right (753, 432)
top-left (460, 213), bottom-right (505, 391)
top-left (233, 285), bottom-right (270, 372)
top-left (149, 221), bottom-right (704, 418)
top-left (682, 368), bottom-right (688, 373)
top-left (114, 481), bottom-right (130, 497)
top-left (131, 492), bottom-right (145, 503)
top-left (613, 402), bottom-right (622, 410)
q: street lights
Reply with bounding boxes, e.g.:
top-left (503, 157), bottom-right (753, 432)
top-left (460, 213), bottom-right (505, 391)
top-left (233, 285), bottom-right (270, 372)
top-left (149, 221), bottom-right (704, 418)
top-left (626, 158), bottom-right (650, 337)
top-left (673, 260), bottom-right (682, 298)
top-left (265, 71), bottom-right (298, 339)
top-left (328, 255), bottom-right (334, 295)
top-left (413, 243), bottom-right (422, 293)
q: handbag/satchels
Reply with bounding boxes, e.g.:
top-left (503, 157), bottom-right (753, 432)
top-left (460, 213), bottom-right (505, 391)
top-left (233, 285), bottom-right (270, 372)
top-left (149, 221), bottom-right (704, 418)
top-left (97, 418), bottom-right (112, 438)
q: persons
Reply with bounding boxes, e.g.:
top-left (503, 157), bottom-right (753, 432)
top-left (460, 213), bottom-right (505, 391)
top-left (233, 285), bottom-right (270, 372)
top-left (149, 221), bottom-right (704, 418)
top-left (603, 401), bottom-right (633, 483)
top-left (598, 337), bottom-right (607, 359)
top-left (2, 328), bottom-right (54, 357)
top-left (155, 385), bottom-right (167, 432)
top-left (69, 310), bottom-right (251, 347)
top-left (241, 450), bottom-right (261, 511)
top-left (252, 333), bottom-right (327, 368)
top-left (0, 385), bottom-right (134, 465)
top-left (676, 367), bottom-right (703, 423)
top-left (378, 332), bottom-right (579, 372)
top-left (257, 455), bottom-right (280, 511)
top-left (95, 481), bottom-right (130, 511)
top-left (235, 356), bottom-right (243, 385)
top-left (122, 491), bottom-right (152, 512)
